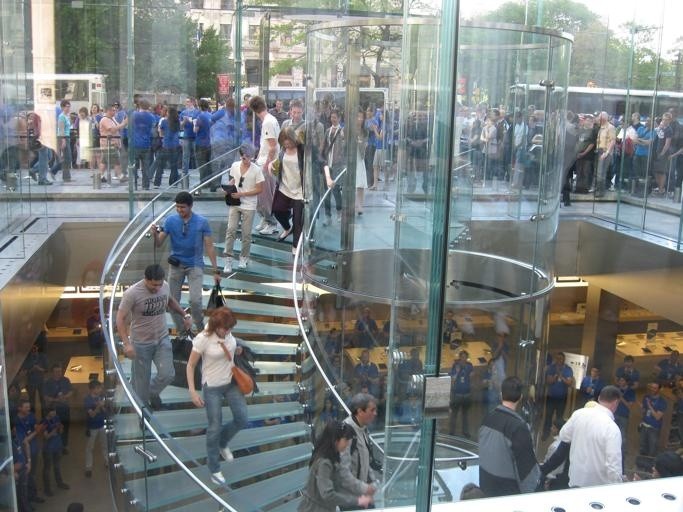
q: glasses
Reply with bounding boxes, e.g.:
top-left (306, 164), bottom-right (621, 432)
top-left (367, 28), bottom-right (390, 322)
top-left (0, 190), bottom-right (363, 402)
top-left (239, 177), bottom-right (244, 187)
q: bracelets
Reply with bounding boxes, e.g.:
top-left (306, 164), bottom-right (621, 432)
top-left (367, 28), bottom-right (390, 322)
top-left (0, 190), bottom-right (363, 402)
top-left (182, 314), bottom-right (191, 319)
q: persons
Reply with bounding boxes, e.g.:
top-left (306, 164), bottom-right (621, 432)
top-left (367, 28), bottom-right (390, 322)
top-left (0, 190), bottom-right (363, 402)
top-left (150, 191), bottom-right (222, 347)
top-left (321, 305), bottom-right (475, 440)
top-left (460, 483), bottom-right (488, 500)
top-left (480, 308), bottom-right (510, 418)
top-left (478, 376), bottom-right (545, 498)
top-left (297, 394), bottom-right (378, 512)
top-left (73, 93), bottom-right (432, 195)
top-left (268, 125), bottom-right (334, 255)
top-left (183, 307), bottom-right (254, 486)
top-left (458, 103), bottom-right (683, 207)
top-left (542, 352), bottom-right (573, 441)
top-left (539, 416), bottom-right (569, 491)
top-left (0, 95), bottom-right (76, 191)
top-left (560, 385), bottom-right (623, 488)
top-left (220, 144), bottom-right (266, 275)
top-left (614, 354), bottom-right (640, 458)
top-left (112, 264), bottom-right (194, 433)
top-left (579, 365), bottom-right (603, 410)
top-left (0, 308), bottom-right (127, 511)
top-left (633, 350), bottom-right (682, 481)
top-left (245, 96), bottom-right (285, 238)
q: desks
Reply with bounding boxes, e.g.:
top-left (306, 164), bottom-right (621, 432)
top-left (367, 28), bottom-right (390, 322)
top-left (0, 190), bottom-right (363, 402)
top-left (42, 325), bottom-right (105, 407)
top-left (500, 305), bottom-right (681, 451)
top-left (310, 305), bottom-right (498, 389)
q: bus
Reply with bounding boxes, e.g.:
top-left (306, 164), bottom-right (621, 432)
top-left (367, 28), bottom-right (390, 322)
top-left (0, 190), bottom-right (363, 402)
top-left (508, 84), bottom-right (683, 124)
top-left (0, 72), bottom-right (110, 115)
top-left (238, 85), bottom-right (390, 111)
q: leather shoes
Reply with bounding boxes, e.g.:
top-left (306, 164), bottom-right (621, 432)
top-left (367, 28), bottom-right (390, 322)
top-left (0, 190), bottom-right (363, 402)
top-left (278, 226), bottom-right (292, 242)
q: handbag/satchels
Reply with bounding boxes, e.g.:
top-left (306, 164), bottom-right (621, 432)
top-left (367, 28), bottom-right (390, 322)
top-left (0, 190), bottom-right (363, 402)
top-left (167, 339), bottom-right (201, 390)
top-left (231, 367), bottom-right (253, 393)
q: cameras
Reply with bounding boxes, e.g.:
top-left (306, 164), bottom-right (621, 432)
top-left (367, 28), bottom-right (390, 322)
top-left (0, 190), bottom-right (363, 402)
top-left (156, 225), bottom-right (166, 233)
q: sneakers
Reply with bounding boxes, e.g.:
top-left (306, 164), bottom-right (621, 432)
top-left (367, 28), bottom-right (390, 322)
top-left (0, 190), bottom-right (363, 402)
top-left (150, 396), bottom-right (160, 409)
top-left (220, 448), bottom-right (232, 462)
top-left (223, 257), bottom-right (232, 274)
top-left (28, 169), bottom-right (36, 180)
top-left (38, 179), bottom-right (50, 185)
top-left (256, 223), bottom-right (277, 234)
top-left (238, 256), bottom-right (248, 268)
top-left (209, 472), bottom-right (225, 484)
